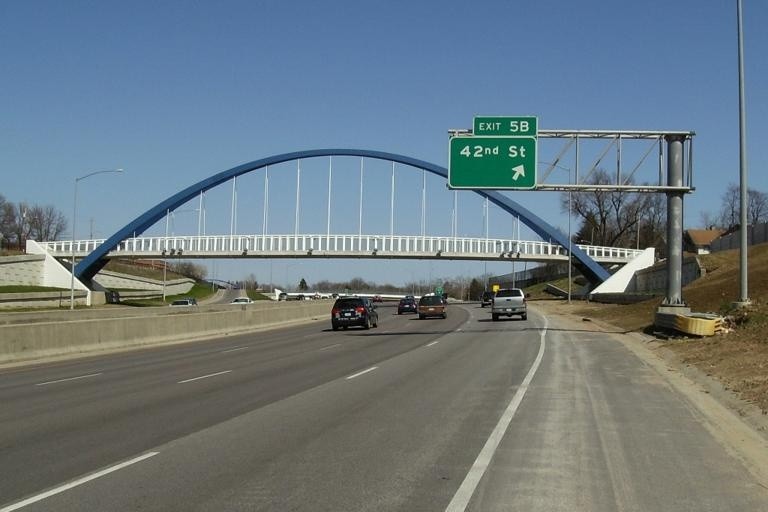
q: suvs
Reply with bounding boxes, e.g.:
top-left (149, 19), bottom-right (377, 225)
top-left (481, 291), bottom-right (497, 307)
top-left (418, 295), bottom-right (448, 320)
top-left (397, 298), bottom-right (417, 316)
top-left (491, 288), bottom-right (527, 321)
top-left (331, 295), bottom-right (379, 331)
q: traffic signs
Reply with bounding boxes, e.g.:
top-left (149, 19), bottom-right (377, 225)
top-left (472, 114), bottom-right (539, 138)
top-left (448, 134), bottom-right (537, 191)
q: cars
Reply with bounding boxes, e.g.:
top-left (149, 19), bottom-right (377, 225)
top-left (372, 296), bottom-right (383, 303)
top-left (169, 299), bottom-right (195, 307)
top-left (229, 297), bottom-right (255, 305)
top-left (278, 291), bottom-right (338, 302)
top-left (232, 284), bottom-right (240, 290)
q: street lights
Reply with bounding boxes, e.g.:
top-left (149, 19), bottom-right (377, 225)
top-left (70, 168), bottom-right (127, 308)
top-left (162, 208), bottom-right (201, 303)
top-left (580, 239), bottom-right (593, 255)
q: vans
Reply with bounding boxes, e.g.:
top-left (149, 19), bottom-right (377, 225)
top-left (405, 296), bottom-right (415, 301)
